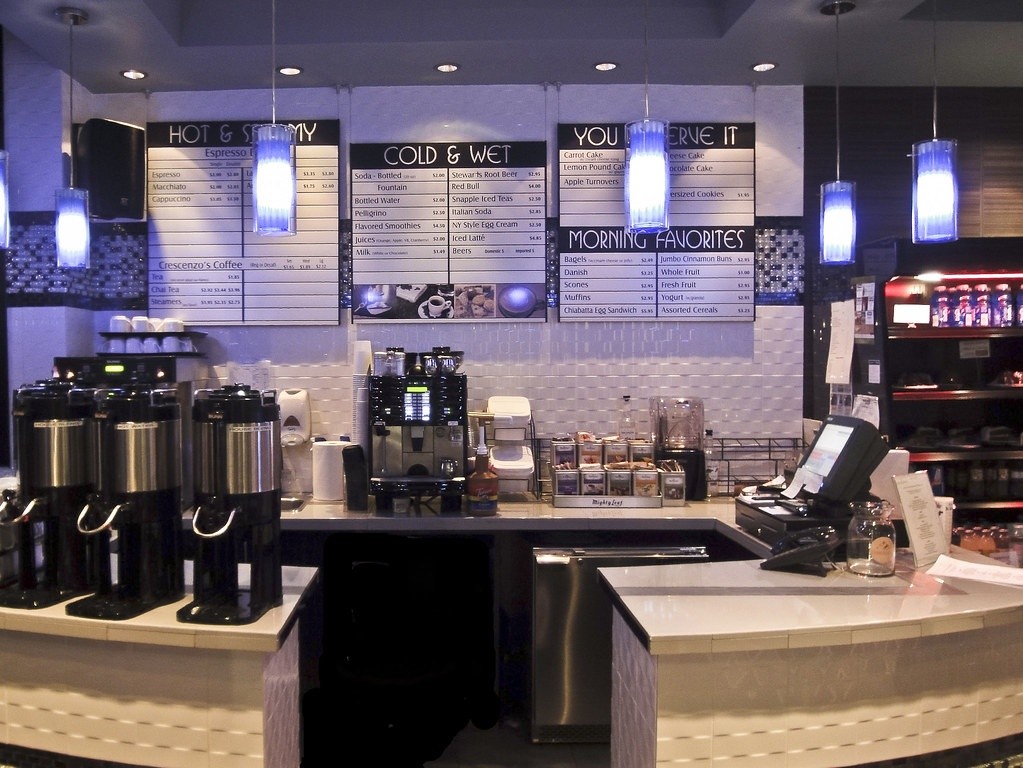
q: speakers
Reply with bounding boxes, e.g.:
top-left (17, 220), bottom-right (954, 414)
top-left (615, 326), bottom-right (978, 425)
top-left (74, 118), bottom-right (146, 220)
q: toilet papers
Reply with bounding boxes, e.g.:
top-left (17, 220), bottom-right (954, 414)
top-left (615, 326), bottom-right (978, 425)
top-left (312, 440), bottom-right (352, 501)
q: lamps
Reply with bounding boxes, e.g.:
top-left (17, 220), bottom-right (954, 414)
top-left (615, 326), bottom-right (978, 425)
top-left (53, 8), bottom-right (100, 269)
top-left (909, 0), bottom-right (960, 243)
top-left (252, 0), bottom-right (299, 238)
top-left (818, 2), bottom-right (859, 268)
top-left (624, 1), bottom-right (669, 235)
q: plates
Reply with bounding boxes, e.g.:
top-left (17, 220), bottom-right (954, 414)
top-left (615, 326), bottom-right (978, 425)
top-left (366, 302), bottom-right (393, 315)
top-left (418, 300), bottom-right (454, 319)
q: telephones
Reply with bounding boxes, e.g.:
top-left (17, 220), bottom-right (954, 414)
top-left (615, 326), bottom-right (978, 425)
top-left (759, 526), bottom-right (846, 571)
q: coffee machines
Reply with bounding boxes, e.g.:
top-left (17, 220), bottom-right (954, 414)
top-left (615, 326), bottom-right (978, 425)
top-left (65, 383), bottom-right (186, 622)
top-left (176, 383), bottom-right (284, 626)
top-left (370, 377), bottom-right (468, 511)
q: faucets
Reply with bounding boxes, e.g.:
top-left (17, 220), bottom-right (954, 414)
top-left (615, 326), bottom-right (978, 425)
top-left (448, 426), bottom-right (457, 442)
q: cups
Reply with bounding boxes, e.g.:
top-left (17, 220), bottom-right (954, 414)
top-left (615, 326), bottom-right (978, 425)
top-left (350, 340), bottom-right (373, 464)
top-left (391, 352), bottom-right (407, 376)
top-left (374, 351), bottom-right (386, 377)
top-left (426, 357), bottom-right (437, 375)
top-left (439, 358), bottom-right (456, 377)
top-left (109, 315), bottom-right (197, 353)
top-left (428, 296), bottom-right (452, 315)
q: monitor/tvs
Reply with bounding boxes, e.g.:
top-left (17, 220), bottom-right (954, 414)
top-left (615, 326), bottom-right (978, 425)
top-left (796, 414), bottom-right (891, 502)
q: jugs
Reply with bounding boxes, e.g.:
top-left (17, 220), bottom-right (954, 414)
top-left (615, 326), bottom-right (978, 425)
top-left (438, 458), bottom-right (458, 478)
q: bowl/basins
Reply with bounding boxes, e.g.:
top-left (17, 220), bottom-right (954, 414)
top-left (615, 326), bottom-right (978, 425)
top-left (0, 377), bottom-right (96, 610)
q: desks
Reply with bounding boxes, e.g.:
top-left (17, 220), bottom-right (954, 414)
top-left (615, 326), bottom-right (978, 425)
top-left (274, 496), bottom-right (781, 558)
top-left (0, 553), bottom-right (320, 768)
top-left (594, 539), bottom-right (1023, 768)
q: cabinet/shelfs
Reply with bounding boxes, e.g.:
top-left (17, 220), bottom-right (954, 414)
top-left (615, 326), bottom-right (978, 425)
top-left (854, 279), bottom-right (1023, 513)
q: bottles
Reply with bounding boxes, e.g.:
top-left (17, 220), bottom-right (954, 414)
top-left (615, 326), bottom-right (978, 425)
top-left (703, 429), bottom-right (719, 497)
top-left (619, 395), bottom-right (635, 441)
top-left (930, 284), bottom-right (1023, 329)
top-left (844, 500), bottom-right (898, 577)
top-left (466, 454), bottom-right (499, 517)
top-left (909, 461), bottom-right (1023, 569)
